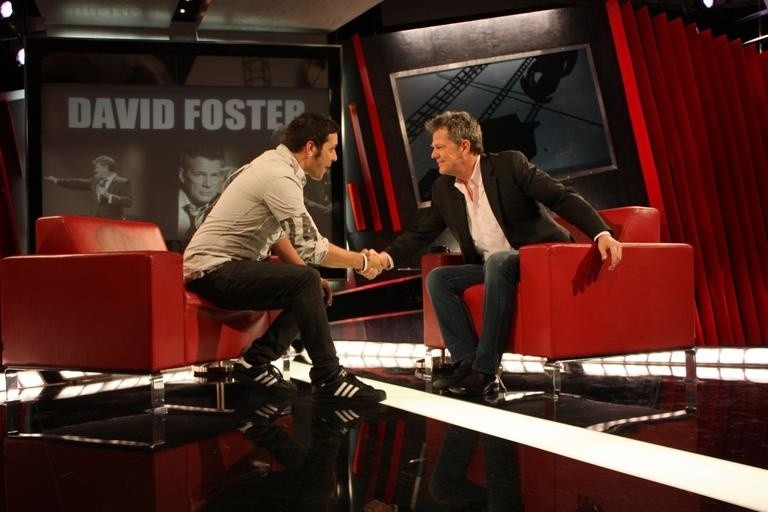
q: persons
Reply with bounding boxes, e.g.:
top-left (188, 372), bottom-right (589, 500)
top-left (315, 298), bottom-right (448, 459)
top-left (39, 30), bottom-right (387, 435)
top-left (213, 403), bottom-right (387, 512)
top-left (428, 424), bottom-right (526, 512)
top-left (371, 111), bottom-right (623, 394)
top-left (155, 142), bottom-right (236, 253)
top-left (42, 154), bottom-right (133, 221)
top-left (181, 114), bottom-right (387, 405)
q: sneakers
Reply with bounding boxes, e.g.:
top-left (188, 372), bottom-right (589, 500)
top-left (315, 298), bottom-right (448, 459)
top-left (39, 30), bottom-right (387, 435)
top-left (227, 399), bottom-right (293, 445)
top-left (309, 366), bottom-right (388, 404)
top-left (230, 355), bottom-right (299, 394)
top-left (312, 405), bottom-right (381, 442)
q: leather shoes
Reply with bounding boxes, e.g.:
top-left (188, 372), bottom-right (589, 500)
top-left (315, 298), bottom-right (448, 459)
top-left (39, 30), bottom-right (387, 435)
top-left (430, 357), bottom-right (501, 399)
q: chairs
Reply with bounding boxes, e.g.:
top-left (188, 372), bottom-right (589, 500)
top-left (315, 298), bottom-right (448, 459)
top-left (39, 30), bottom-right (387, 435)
top-left (0, 214), bottom-right (284, 452)
top-left (413, 204), bottom-right (699, 436)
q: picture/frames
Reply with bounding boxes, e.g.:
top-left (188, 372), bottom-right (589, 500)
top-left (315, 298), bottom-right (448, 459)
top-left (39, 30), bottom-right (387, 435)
top-left (389, 43), bottom-right (620, 209)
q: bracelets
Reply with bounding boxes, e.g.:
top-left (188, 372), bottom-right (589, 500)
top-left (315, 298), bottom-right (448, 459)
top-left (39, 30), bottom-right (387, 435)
top-left (357, 251), bottom-right (368, 272)
